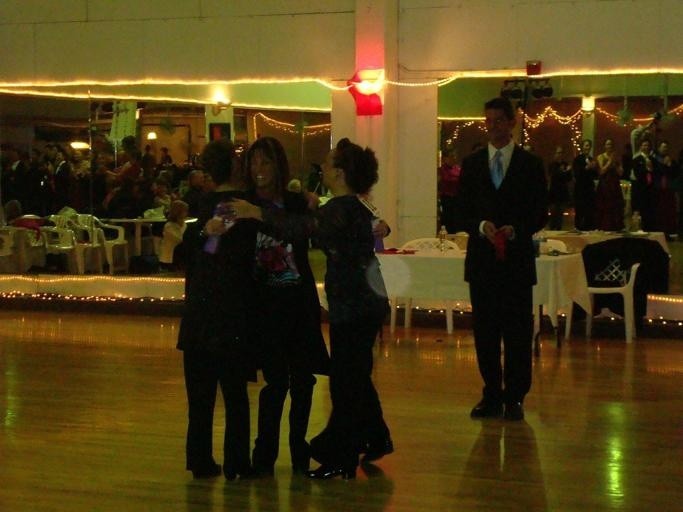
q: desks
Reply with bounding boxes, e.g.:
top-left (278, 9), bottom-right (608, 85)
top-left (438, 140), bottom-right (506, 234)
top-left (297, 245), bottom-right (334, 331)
top-left (470, 223), bottom-right (668, 255)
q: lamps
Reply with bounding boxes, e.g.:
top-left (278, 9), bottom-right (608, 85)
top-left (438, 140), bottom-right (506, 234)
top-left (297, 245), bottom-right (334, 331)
top-left (580, 94), bottom-right (596, 113)
top-left (208, 85), bottom-right (233, 117)
top-left (352, 76), bottom-right (387, 97)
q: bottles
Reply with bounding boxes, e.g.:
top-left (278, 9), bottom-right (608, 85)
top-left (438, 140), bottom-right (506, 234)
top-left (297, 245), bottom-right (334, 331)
top-left (438, 224), bottom-right (448, 256)
top-left (562, 207), bottom-right (575, 232)
top-left (630, 210), bottom-right (642, 231)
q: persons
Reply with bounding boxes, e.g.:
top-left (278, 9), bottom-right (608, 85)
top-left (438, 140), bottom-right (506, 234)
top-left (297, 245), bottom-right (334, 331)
top-left (172, 140), bottom-right (391, 475)
top-left (436, 138), bottom-right (683, 243)
top-left (221, 139), bottom-right (394, 481)
top-left (177, 137), bottom-right (322, 481)
top-left (454, 98), bottom-right (548, 421)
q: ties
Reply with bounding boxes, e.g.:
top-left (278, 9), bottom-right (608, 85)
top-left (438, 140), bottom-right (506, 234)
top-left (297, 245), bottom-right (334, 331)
top-left (490, 151), bottom-right (503, 188)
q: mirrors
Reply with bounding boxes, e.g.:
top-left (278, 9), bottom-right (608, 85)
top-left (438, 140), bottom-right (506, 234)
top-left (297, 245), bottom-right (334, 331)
top-left (433, 68), bottom-right (683, 302)
top-left (0, 74), bottom-right (336, 285)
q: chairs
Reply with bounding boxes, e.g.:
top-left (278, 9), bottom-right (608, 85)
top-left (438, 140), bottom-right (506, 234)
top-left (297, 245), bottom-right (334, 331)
top-left (374, 234), bottom-right (663, 353)
top-left (0, 199), bottom-right (203, 278)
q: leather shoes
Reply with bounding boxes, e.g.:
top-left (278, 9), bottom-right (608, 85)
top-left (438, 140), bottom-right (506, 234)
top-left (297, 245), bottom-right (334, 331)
top-left (503, 401), bottom-right (524, 420)
top-left (471, 398), bottom-right (504, 419)
top-left (191, 439), bottom-right (394, 479)
